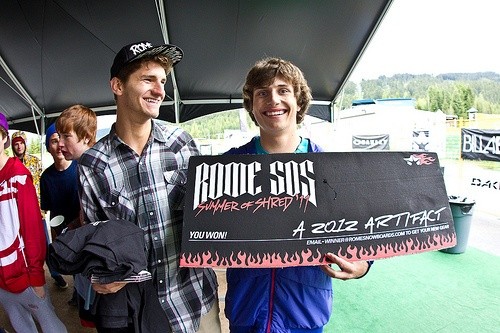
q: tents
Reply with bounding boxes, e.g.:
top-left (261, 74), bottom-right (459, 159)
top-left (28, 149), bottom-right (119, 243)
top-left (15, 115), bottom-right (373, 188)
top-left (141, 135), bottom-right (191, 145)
top-left (297, 99), bottom-right (446, 156)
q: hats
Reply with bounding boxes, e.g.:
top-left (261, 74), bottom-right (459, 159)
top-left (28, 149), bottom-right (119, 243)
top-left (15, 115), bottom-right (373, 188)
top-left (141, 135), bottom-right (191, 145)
top-left (11, 131), bottom-right (27, 157)
top-left (110, 40), bottom-right (184, 80)
top-left (45, 122), bottom-right (58, 153)
top-left (0, 113), bottom-right (10, 149)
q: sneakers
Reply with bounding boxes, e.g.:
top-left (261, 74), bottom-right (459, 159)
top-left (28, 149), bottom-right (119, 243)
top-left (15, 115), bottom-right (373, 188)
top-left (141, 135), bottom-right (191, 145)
top-left (53, 275), bottom-right (68, 289)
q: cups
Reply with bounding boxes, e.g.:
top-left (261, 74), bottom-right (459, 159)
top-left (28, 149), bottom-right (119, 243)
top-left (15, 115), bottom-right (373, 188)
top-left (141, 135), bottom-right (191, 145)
top-left (49, 216), bottom-right (67, 234)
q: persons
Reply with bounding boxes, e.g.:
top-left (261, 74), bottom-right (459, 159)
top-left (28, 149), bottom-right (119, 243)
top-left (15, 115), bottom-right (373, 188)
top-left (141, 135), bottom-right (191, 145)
top-left (0, 105), bottom-right (97, 333)
top-left (78, 40), bottom-right (221, 333)
top-left (219, 57), bottom-right (374, 333)
